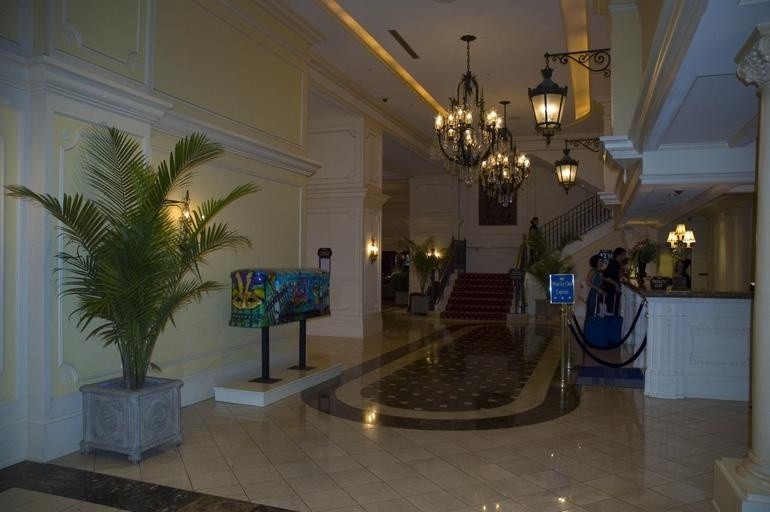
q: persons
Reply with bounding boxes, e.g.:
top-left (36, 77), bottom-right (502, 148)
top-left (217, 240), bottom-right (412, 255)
top-left (529, 217), bottom-right (542, 264)
top-left (586, 247), bottom-right (628, 318)
top-left (673, 260), bottom-right (690, 290)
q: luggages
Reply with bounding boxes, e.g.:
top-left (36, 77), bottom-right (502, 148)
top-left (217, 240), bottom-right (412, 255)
top-left (586, 292), bottom-right (609, 348)
top-left (606, 292), bottom-right (626, 345)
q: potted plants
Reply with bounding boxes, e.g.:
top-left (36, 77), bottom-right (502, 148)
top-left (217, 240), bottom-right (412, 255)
top-left (518, 230), bottom-right (584, 327)
top-left (2, 119), bottom-right (260, 466)
top-left (629, 238), bottom-right (663, 278)
top-left (402, 234), bottom-right (453, 315)
top-left (392, 272), bottom-right (409, 306)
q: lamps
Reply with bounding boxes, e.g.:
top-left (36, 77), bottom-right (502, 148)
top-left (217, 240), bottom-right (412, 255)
top-left (666, 224), bottom-right (696, 290)
top-left (428, 34), bottom-right (611, 207)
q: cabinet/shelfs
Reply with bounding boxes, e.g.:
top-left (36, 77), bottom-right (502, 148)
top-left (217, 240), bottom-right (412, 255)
top-left (382, 251), bottom-right (397, 273)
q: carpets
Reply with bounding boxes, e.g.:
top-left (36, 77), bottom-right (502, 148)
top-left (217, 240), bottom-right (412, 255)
top-left (574, 366), bottom-right (645, 388)
